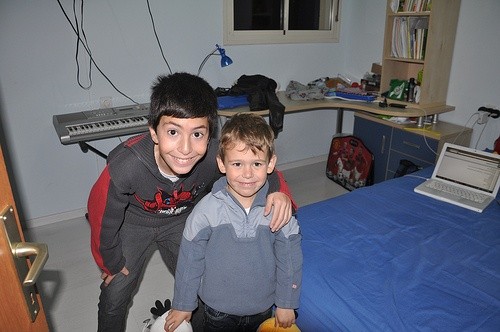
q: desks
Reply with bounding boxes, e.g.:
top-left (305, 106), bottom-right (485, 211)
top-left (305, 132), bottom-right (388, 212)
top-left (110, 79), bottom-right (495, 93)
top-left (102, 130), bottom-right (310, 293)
top-left (290, 167), bottom-right (500, 332)
top-left (215, 88), bottom-right (455, 135)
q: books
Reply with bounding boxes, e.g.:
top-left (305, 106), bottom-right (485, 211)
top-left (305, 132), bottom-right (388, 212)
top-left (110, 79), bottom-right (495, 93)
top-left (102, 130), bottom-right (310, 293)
top-left (391, 17), bottom-right (428, 60)
top-left (392, 0), bottom-right (432, 12)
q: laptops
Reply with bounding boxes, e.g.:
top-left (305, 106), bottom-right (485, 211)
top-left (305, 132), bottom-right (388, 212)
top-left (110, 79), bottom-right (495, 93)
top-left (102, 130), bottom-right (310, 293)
top-left (414, 142), bottom-right (500, 213)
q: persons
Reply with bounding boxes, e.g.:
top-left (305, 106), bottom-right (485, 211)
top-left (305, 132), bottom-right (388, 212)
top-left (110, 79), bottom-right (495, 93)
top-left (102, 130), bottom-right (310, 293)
top-left (167, 114), bottom-right (303, 332)
top-left (87, 73), bottom-right (298, 332)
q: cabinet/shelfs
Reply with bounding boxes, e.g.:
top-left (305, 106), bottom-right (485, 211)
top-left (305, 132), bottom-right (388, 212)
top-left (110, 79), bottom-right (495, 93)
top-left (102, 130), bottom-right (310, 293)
top-left (378, 0), bottom-right (462, 108)
top-left (354, 111), bottom-right (473, 186)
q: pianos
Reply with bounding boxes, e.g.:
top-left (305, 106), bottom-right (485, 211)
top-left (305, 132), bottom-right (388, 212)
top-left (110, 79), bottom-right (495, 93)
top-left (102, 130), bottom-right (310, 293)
top-left (53, 103), bottom-right (150, 159)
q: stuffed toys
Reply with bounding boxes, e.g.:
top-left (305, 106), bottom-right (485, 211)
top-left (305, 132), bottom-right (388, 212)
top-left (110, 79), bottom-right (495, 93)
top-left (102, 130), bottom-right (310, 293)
top-left (257, 317), bottom-right (301, 332)
top-left (143, 299), bottom-right (193, 332)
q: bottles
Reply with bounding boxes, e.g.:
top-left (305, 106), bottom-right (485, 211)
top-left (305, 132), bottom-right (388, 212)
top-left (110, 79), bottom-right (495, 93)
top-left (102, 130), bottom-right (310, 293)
top-left (413, 81), bottom-right (421, 103)
top-left (408, 77), bottom-right (415, 102)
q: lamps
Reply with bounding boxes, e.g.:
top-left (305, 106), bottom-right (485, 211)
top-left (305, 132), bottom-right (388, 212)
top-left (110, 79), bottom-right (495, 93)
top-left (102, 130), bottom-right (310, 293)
top-left (197, 43), bottom-right (233, 75)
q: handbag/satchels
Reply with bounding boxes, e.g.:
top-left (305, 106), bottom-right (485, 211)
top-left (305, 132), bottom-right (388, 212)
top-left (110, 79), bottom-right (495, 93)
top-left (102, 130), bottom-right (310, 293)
top-left (325, 135), bottom-right (375, 192)
